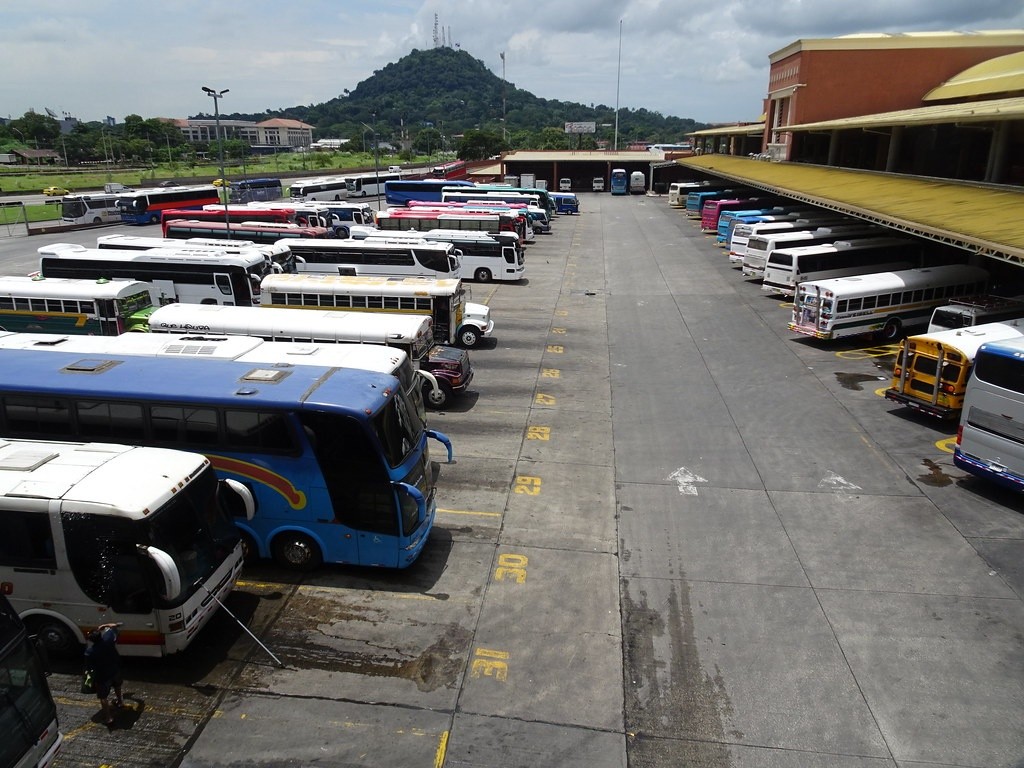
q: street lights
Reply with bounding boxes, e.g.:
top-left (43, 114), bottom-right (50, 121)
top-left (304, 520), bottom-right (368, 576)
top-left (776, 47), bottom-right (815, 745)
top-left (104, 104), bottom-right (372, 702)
top-left (201, 86), bottom-right (231, 242)
top-left (499, 50), bottom-right (507, 138)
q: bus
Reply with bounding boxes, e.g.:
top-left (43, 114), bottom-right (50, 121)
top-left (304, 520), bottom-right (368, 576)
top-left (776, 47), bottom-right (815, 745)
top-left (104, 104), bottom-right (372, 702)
top-left (882, 318), bottom-right (1024, 421)
top-left (0, 160), bottom-right (581, 462)
top-left (761, 237), bottom-right (926, 297)
top-left (0, 347), bottom-right (457, 585)
top-left (953, 336), bottom-right (1024, 495)
top-left (0, 589), bottom-right (91, 768)
top-left (611, 168), bottom-right (646, 196)
top-left (592, 178), bottom-right (605, 191)
top-left (667, 177), bottom-right (890, 281)
top-left (559, 178), bottom-right (572, 191)
top-left (0, 434), bottom-right (256, 659)
top-left (927, 293), bottom-right (1024, 337)
top-left (787, 263), bottom-right (997, 342)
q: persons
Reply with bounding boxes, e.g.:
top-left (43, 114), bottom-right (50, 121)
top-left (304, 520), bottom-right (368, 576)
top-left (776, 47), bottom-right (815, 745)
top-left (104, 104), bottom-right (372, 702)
top-left (286, 216), bottom-right (308, 226)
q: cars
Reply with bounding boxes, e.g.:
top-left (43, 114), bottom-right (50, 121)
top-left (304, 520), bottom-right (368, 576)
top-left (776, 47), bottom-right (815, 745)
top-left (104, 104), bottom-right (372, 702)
top-left (212, 178), bottom-right (231, 187)
top-left (160, 181), bottom-right (179, 188)
top-left (43, 185), bottom-right (70, 197)
